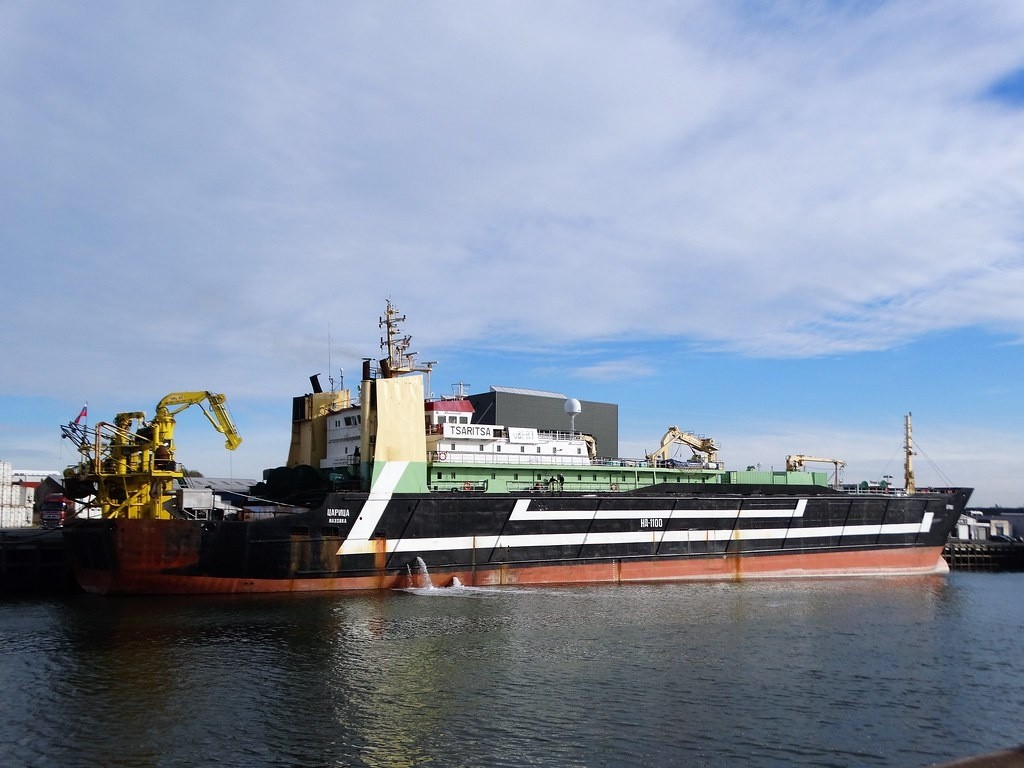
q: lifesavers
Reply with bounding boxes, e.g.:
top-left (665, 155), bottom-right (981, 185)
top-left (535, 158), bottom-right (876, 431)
top-left (439, 452), bottom-right (447, 461)
top-left (464, 482), bottom-right (471, 491)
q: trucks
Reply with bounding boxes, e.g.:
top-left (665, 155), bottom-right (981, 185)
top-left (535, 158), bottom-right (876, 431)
top-left (39, 493), bottom-right (102, 530)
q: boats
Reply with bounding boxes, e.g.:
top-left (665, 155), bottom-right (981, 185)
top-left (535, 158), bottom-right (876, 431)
top-left (64, 293), bottom-right (976, 595)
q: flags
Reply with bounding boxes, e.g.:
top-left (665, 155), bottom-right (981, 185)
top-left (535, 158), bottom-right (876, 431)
top-left (75, 402), bottom-right (88, 425)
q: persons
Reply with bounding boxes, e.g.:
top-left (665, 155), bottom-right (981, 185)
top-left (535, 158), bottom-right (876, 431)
top-left (549, 476), bottom-right (558, 491)
top-left (560, 473), bottom-right (564, 490)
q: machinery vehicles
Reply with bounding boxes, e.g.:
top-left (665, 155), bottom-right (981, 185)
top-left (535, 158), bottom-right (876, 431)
top-left (58, 390), bottom-right (243, 520)
